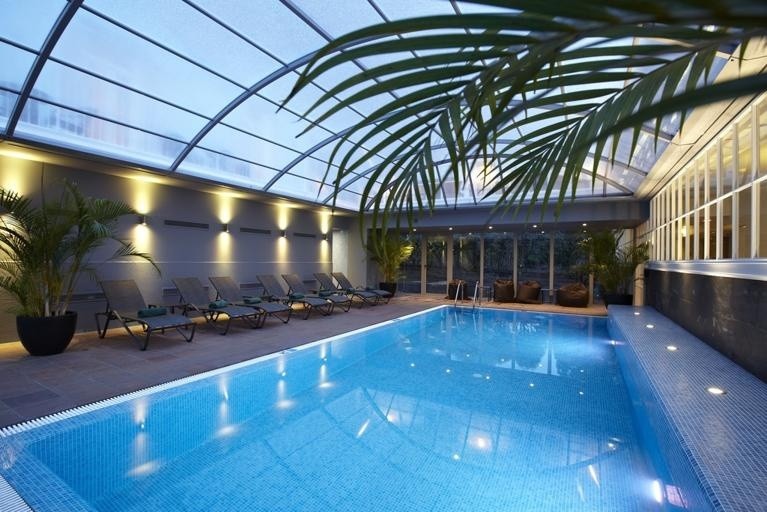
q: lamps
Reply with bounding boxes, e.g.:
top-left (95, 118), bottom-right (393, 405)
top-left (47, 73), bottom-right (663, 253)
top-left (137, 214), bottom-right (149, 228)
top-left (221, 223), bottom-right (229, 233)
top-left (320, 233), bottom-right (329, 241)
top-left (278, 229), bottom-right (287, 238)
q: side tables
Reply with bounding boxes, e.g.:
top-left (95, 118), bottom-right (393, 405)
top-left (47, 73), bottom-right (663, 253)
top-left (243, 295), bottom-right (280, 319)
top-left (148, 303), bottom-right (190, 335)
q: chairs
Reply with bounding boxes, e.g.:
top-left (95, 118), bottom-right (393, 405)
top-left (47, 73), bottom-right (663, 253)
top-left (280, 274), bottom-right (332, 320)
top-left (171, 277), bottom-right (262, 335)
top-left (208, 276), bottom-right (293, 329)
top-left (313, 273), bottom-right (394, 316)
top-left (94, 279), bottom-right (197, 351)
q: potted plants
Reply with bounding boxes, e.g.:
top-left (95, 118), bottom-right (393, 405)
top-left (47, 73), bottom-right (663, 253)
top-left (362, 236), bottom-right (418, 300)
top-left (0, 172), bottom-right (168, 358)
top-left (569, 219), bottom-right (654, 310)
